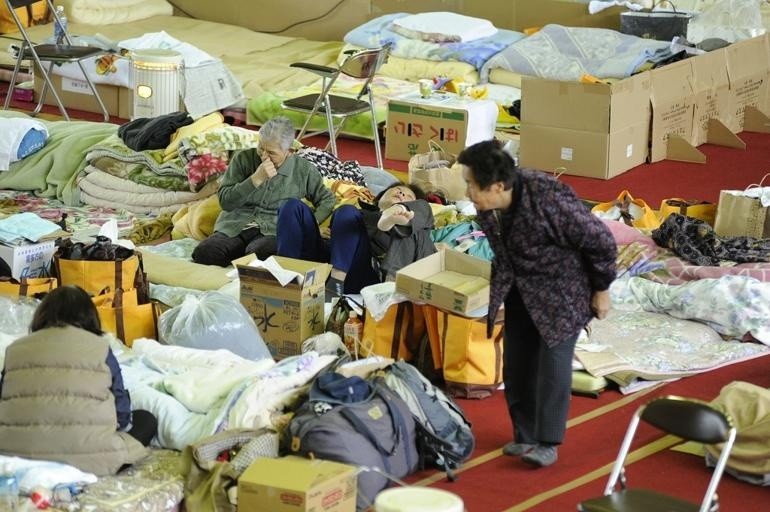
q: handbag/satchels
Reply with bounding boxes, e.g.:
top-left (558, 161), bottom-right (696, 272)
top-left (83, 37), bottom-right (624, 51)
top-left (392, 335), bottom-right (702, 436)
top-left (280, 353), bottom-right (474, 511)
top-left (1, 243), bottom-right (161, 347)
top-left (591, 190), bottom-right (660, 229)
top-left (183, 428), bottom-right (277, 512)
top-left (358, 293), bottom-right (426, 365)
top-left (423, 303), bottom-right (506, 400)
top-left (714, 190), bottom-right (770, 240)
top-left (619, 10), bottom-right (689, 44)
top-left (407, 149), bottom-right (466, 202)
top-left (660, 197), bottom-right (716, 231)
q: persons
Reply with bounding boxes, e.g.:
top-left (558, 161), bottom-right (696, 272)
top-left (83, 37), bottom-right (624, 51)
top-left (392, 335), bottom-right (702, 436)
top-left (192, 115), bottom-right (335, 271)
top-left (274, 184), bottom-right (435, 298)
top-left (0, 287), bottom-right (157, 481)
top-left (459, 140), bottom-right (617, 468)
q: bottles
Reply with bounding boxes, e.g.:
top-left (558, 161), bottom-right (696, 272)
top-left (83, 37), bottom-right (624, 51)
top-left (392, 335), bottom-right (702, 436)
top-left (344, 311), bottom-right (364, 360)
top-left (0, 464), bottom-right (89, 512)
top-left (54, 5), bottom-right (67, 47)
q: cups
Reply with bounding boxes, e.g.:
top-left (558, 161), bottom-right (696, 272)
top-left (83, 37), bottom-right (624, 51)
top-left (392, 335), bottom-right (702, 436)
top-left (418, 79), bottom-right (434, 99)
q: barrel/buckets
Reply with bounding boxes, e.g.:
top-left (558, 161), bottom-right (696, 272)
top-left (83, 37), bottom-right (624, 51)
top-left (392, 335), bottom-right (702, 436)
top-left (372, 486), bottom-right (464, 512)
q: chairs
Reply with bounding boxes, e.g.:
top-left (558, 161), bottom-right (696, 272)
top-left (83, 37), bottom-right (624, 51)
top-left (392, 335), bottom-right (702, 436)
top-left (577, 395), bottom-right (736, 512)
top-left (281, 43), bottom-right (389, 171)
top-left (2, 0), bottom-right (109, 123)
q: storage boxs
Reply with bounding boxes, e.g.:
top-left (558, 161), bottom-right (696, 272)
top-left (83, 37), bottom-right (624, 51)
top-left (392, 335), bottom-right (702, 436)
top-left (385, 91), bottom-right (499, 165)
top-left (237, 454), bottom-right (359, 511)
top-left (116, 84), bottom-right (184, 120)
top-left (233, 253), bottom-right (333, 363)
top-left (394, 248), bottom-right (491, 312)
top-left (31, 62), bottom-right (116, 118)
top-left (0, 241), bottom-right (54, 280)
top-left (518, 32), bottom-right (766, 180)
